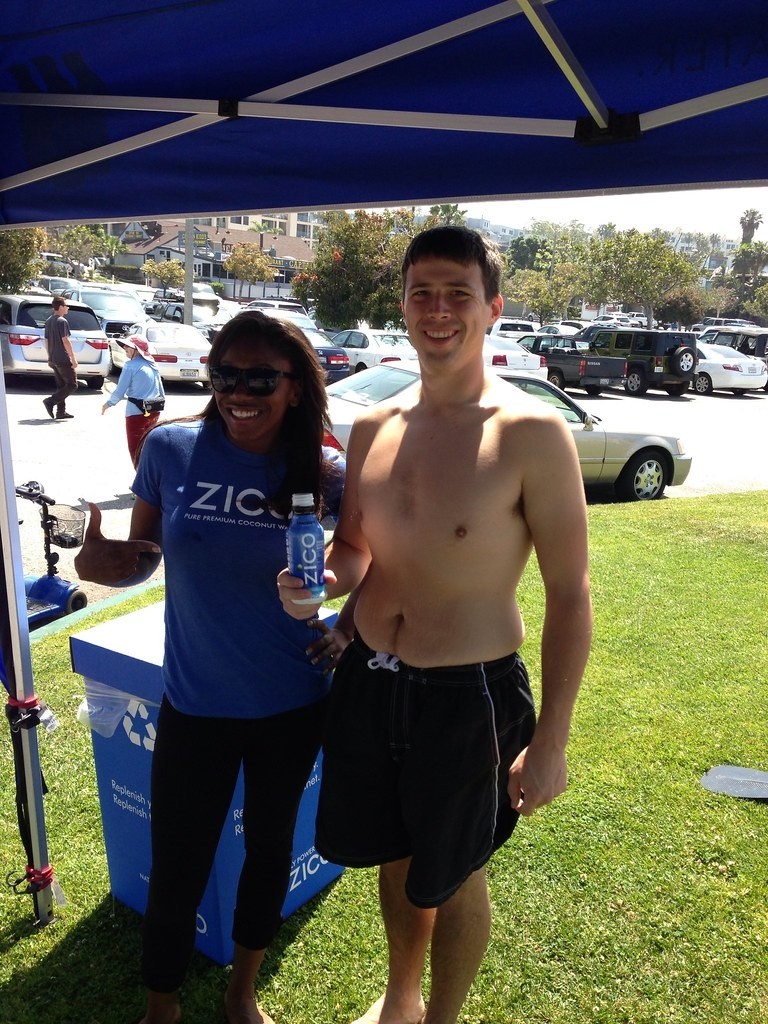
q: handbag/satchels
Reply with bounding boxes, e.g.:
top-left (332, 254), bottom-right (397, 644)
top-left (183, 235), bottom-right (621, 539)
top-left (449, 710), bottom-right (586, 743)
top-left (138, 399), bottom-right (165, 415)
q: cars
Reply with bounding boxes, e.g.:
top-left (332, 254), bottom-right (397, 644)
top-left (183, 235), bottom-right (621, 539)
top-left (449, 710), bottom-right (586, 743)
top-left (483, 335), bottom-right (550, 380)
top-left (330, 327), bottom-right (421, 375)
top-left (488, 310), bottom-right (670, 335)
top-left (34, 254), bottom-right (73, 271)
top-left (60, 289), bottom-right (157, 336)
top-left (690, 317), bottom-right (768, 366)
top-left (108, 322), bottom-right (214, 391)
top-left (694, 342), bottom-right (768, 395)
top-left (20, 273), bottom-right (311, 320)
top-left (323, 362), bottom-right (693, 502)
top-left (205, 307), bottom-right (351, 383)
top-left (0, 294), bottom-right (110, 389)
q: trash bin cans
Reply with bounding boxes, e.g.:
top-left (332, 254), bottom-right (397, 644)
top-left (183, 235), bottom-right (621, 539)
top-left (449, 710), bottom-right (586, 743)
top-left (67, 596), bottom-right (347, 967)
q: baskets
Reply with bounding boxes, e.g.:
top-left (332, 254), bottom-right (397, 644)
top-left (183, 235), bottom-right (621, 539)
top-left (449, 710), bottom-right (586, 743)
top-left (39, 503), bottom-right (86, 549)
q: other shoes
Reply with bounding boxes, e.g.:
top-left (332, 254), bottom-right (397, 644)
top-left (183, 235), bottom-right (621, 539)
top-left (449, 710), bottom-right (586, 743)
top-left (129, 493), bottom-right (138, 501)
top-left (43, 397), bottom-right (56, 419)
top-left (56, 411), bottom-right (73, 420)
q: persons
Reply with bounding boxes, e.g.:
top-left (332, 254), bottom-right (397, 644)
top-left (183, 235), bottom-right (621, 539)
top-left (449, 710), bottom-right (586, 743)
top-left (277, 226), bottom-right (594, 1024)
top-left (102, 335), bottom-right (166, 499)
top-left (75, 312), bottom-right (374, 1024)
top-left (43, 296), bottom-right (78, 419)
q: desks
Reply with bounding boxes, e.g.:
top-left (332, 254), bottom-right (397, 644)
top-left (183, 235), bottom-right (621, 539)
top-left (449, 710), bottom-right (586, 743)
top-left (69, 598), bottom-right (345, 966)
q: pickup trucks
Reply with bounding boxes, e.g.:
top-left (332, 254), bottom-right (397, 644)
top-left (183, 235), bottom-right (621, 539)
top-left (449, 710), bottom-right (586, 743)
top-left (517, 334), bottom-right (628, 396)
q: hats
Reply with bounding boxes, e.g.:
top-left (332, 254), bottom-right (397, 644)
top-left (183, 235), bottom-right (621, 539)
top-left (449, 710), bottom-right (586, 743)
top-left (115, 336), bottom-right (154, 365)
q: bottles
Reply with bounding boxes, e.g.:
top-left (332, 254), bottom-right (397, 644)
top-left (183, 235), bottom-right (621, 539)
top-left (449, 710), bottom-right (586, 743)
top-left (285, 493), bottom-right (328, 605)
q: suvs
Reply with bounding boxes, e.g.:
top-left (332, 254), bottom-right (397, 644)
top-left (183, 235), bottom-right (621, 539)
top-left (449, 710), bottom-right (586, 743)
top-left (570, 329), bottom-right (698, 398)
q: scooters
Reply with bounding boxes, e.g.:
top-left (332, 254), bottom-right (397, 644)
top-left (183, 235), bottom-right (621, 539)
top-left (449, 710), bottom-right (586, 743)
top-left (14, 481), bottom-right (87, 626)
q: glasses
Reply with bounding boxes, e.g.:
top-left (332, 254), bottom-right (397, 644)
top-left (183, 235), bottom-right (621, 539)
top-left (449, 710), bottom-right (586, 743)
top-left (62, 304), bottom-right (69, 308)
top-left (206, 361), bottom-right (291, 399)
